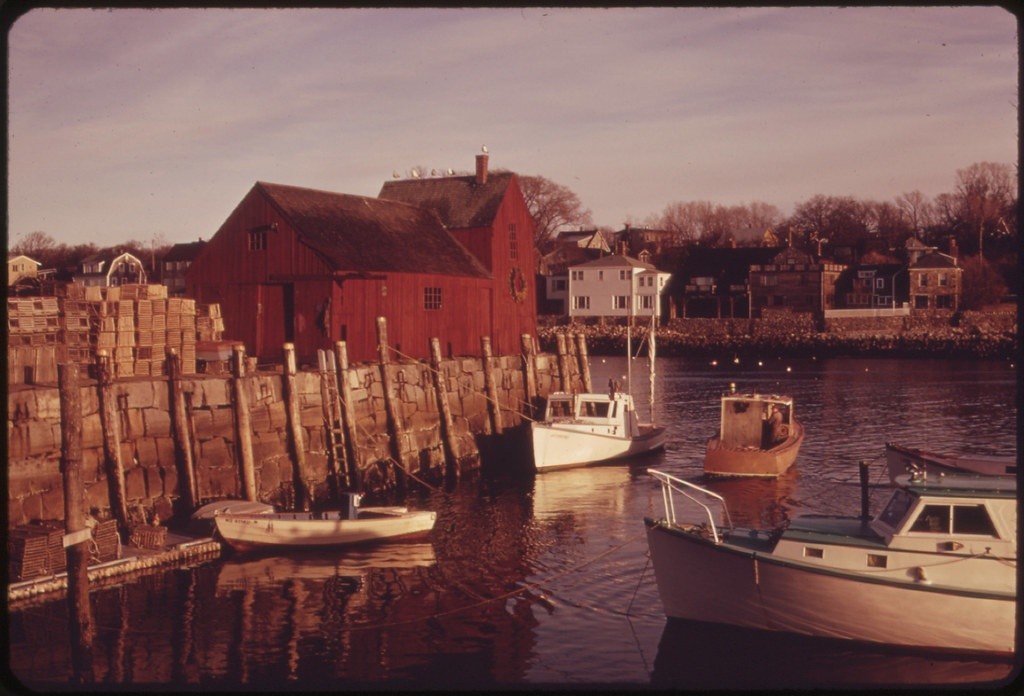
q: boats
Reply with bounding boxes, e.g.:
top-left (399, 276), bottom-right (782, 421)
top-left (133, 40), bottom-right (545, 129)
top-left (700, 385), bottom-right (803, 478)
top-left (645, 457), bottom-right (1018, 661)
top-left (208, 494), bottom-right (441, 553)
top-left (883, 439), bottom-right (1018, 481)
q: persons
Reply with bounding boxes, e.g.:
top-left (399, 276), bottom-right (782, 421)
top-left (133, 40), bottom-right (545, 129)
top-left (768, 406), bottom-right (783, 445)
top-left (608, 379), bottom-right (619, 400)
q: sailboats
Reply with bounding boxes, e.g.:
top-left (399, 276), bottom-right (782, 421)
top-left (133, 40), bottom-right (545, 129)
top-left (527, 290), bottom-right (669, 464)
